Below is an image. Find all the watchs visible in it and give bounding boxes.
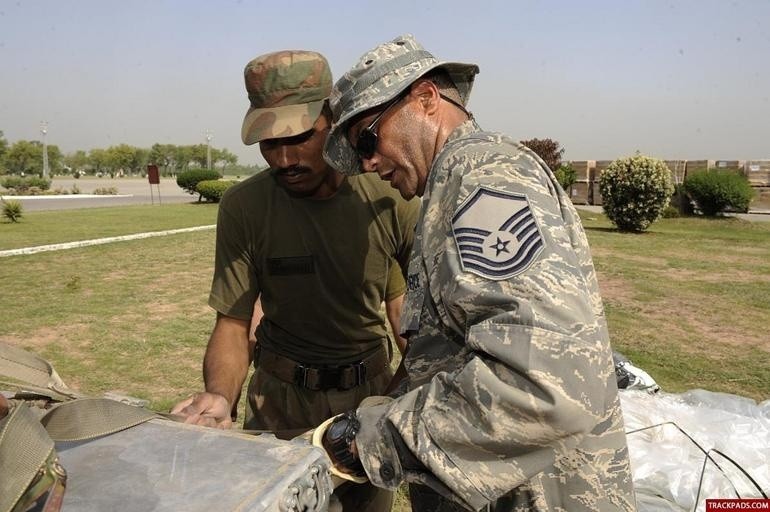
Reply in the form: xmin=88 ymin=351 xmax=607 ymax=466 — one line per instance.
xmin=327 ymin=408 xmax=366 ymax=477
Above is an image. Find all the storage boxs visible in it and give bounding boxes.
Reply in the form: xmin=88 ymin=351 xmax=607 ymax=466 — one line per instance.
xmin=560 ymin=159 xmax=770 ymax=214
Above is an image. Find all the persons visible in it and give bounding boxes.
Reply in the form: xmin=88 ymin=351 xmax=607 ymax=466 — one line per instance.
xmin=167 ymin=51 xmax=423 ymax=512
xmin=290 ymin=34 xmax=634 ymax=512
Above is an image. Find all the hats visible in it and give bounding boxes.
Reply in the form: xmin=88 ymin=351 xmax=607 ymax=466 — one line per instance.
xmin=322 ymin=34 xmax=479 ymax=177
xmin=241 ymin=51 xmax=332 ymax=145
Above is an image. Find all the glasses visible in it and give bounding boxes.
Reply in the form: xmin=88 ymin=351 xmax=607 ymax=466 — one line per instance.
xmin=356 ymin=88 xmax=411 ymax=160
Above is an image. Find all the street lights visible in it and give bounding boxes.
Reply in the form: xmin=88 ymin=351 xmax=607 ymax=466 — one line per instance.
xmin=206 ymin=128 xmax=212 ymax=171
xmin=40 ymin=120 xmax=50 ymax=181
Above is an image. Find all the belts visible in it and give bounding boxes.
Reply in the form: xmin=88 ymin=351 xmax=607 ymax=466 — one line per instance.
xmin=257 ymin=346 xmax=390 ymax=391
